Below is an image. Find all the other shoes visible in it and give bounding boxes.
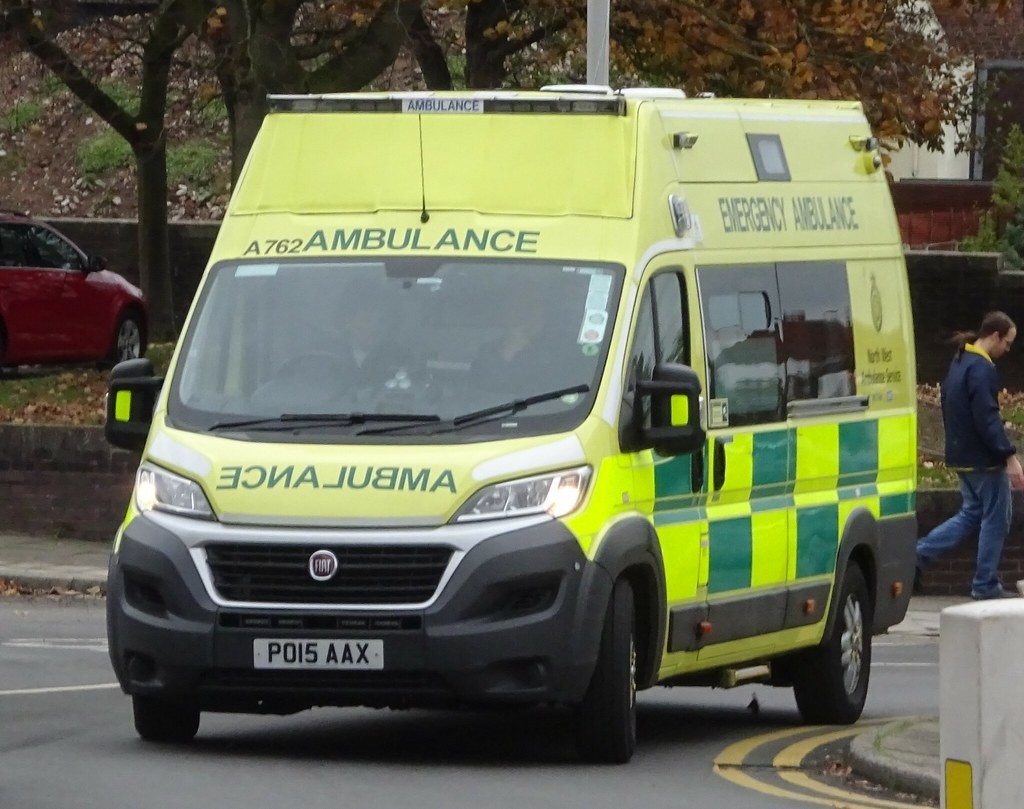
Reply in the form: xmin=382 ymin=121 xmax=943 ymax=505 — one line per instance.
xmin=913 ymin=566 xmax=926 ymax=593
xmin=971 ymin=589 xmax=1019 ymax=600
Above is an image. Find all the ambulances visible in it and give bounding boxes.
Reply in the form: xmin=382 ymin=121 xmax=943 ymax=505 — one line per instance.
xmin=104 ymin=80 xmax=922 ymax=764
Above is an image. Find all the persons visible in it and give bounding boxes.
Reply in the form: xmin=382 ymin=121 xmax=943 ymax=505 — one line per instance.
xmin=333 ymin=304 xmax=408 ymax=395
xmin=916 ymin=312 xmax=1024 ymax=600
xmin=470 ymin=281 xmax=558 ymax=403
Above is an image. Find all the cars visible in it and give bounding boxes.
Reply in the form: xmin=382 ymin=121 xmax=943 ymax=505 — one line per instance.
xmin=0 ymin=206 xmax=146 ymax=374
